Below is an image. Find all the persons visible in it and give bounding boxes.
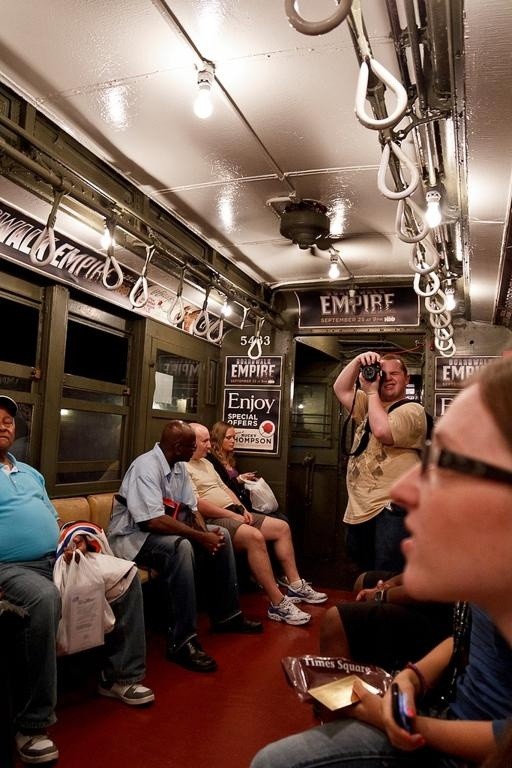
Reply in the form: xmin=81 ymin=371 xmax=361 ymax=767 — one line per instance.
xmin=184 ymin=421 xmax=330 ymax=628
xmin=105 ymin=418 xmax=265 ymax=674
xmin=321 ymin=568 xmax=471 ymax=666
xmin=203 ymin=420 xmax=292 ymax=592
xmin=332 ymin=349 xmax=430 ymax=577
xmin=248 ymin=597 xmax=511 ymax=767
xmin=2 ymin=394 xmax=159 ymax=767
xmin=383 ymin=356 xmax=512 ymax=644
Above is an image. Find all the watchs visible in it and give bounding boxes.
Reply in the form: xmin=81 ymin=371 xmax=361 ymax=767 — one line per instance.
xmin=373 ymin=587 xmax=387 ymax=602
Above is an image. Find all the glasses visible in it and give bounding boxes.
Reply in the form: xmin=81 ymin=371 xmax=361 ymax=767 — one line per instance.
xmin=182 ymin=444 xmax=197 ymax=452
xmin=419 ymin=438 xmax=512 ymax=490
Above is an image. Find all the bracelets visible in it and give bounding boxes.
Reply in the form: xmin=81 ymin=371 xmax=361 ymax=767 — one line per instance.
xmin=405 ymin=660 xmax=428 ymax=697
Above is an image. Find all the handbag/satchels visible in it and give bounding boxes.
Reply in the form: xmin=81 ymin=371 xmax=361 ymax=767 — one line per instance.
xmin=233 ymin=476 xmax=253 ymax=512
xmin=176 ymin=502 xmax=202 ymax=531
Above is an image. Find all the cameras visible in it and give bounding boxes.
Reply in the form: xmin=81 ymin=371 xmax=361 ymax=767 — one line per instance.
xmin=358 ymin=363 xmax=381 ymax=386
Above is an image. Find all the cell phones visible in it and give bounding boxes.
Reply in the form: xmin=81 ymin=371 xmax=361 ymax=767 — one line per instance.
xmin=390 ymin=681 xmax=414 ymax=735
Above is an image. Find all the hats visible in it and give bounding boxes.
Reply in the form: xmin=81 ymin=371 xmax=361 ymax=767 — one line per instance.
xmin=0 ymin=393 xmax=19 ymax=418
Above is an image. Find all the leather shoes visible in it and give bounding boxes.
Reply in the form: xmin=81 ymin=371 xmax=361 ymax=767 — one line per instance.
xmin=209 ymin=618 xmax=264 ymax=634
xmin=167 ymin=637 xmax=218 ymax=673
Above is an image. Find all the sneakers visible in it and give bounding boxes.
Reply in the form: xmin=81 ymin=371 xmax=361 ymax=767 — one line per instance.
xmin=97 ymin=670 xmax=155 ymax=705
xmin=15 ymin=731 xmax=59 ymax=764
xmin=285 ymin=578 xmax=328 ymax=604
xmin=267 ymin=594 xmax=312 ymax=625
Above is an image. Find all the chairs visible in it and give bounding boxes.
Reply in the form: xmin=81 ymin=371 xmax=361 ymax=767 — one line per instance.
xmin=47 ymin=491 xmax=161 ymax=611
